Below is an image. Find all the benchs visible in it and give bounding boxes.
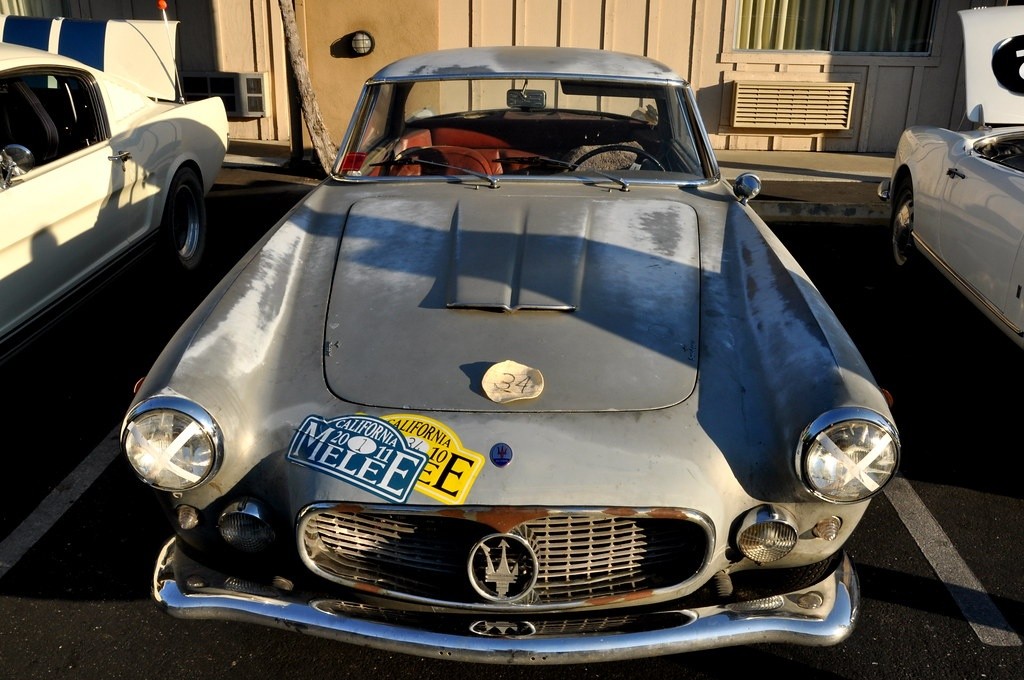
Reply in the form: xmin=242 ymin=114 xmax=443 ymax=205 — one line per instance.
xmin=393 ymin=118 xmax=660 ymax=174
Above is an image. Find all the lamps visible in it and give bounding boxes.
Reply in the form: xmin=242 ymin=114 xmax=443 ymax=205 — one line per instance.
xmin=352 ymin=30 xmax=375 ymax=55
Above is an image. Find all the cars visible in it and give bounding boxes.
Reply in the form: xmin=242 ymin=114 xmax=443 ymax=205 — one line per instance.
xmin=875 ymin=4 xmax=1024 ymax=357
xmin=0 ymin=41 xmax=232 ymax=350
xmin=118 ymin=38 xmax=903 ymax=670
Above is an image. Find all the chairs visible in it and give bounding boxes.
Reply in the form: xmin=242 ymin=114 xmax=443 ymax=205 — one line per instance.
xmin=565 ymin=141 xmax=645 ymax=172
xmin=390 ymin=146 xmax=492 ymax=176
